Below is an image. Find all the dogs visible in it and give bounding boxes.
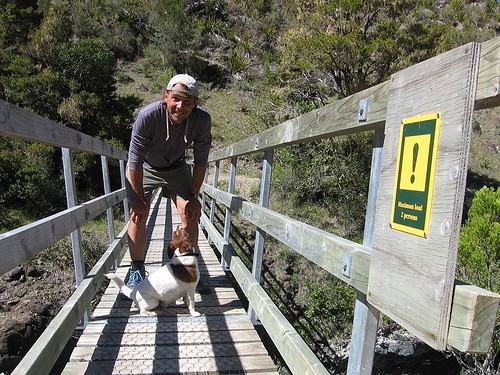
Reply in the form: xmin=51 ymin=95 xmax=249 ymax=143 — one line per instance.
xmin=103 ymin=223 xmax=201 ymax=317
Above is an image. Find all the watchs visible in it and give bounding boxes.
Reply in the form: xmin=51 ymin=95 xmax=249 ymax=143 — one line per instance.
xmin=190 ymin=192 xmax=198 ymax=198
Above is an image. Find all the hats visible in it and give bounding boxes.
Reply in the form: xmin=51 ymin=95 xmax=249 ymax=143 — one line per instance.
xmin=166 ymin=74 xmax=200 ymax=99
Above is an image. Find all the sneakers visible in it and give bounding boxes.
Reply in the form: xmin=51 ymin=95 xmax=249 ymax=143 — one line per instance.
xmin=198 ymin=277 xmax=212 ymax=295
xmin=121 ymin=267 xmax=145 ymax=302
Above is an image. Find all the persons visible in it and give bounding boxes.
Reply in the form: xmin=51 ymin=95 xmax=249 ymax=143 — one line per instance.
xmin=123 ymin=75 xmax=212 ymax=293
